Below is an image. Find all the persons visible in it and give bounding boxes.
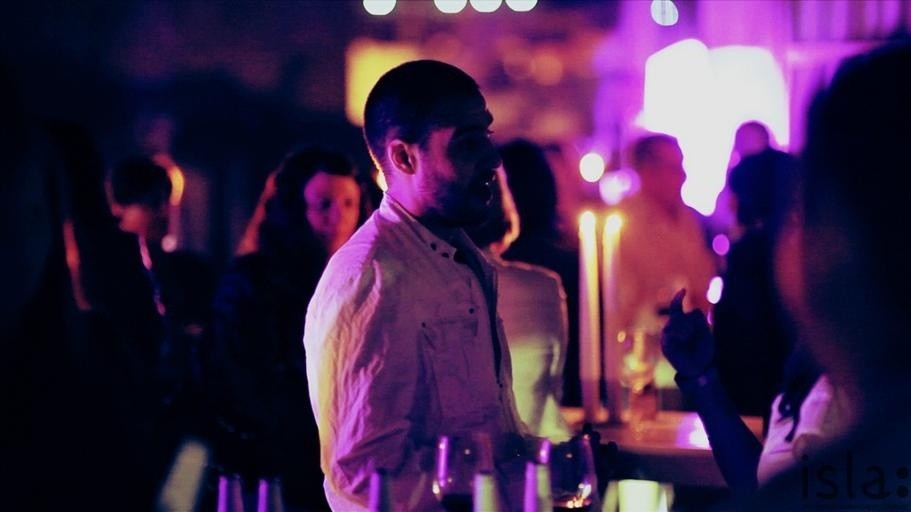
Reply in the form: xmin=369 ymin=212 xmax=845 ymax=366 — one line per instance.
xmin=2 ymin=21 xmax=908 ymax=510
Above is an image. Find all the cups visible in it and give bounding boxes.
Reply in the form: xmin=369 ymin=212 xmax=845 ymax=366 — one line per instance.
xmin=433 ymin=431 xmax=493 ymax=511
xmin=213 ymin=474 xmax=285 ymax=512
xmin=523 ymin=432 xmax=600 ymax=512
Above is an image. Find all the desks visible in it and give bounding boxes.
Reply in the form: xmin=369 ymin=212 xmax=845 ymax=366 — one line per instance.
xmin=555 ymin=404 xmax=766 ymax=512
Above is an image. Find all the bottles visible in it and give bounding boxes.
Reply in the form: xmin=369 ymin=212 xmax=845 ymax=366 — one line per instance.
xmin=618 ymin=325 xmax=657 ymax=423
